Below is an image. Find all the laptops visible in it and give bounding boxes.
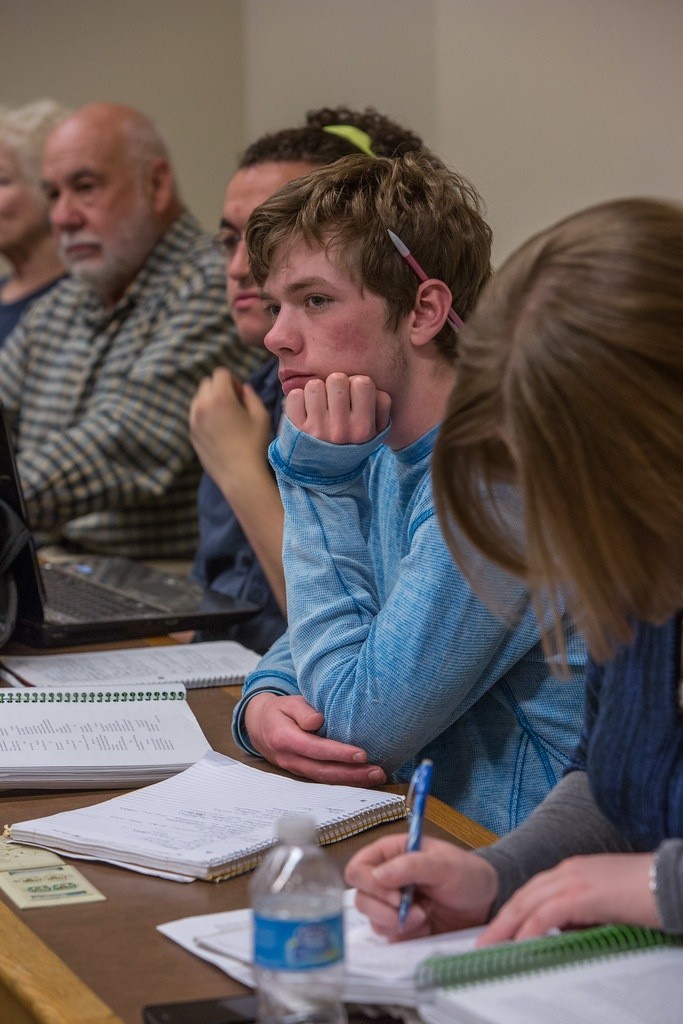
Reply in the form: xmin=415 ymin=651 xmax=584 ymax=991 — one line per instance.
xmin=0 ymin=408 xmax=262 ymax=650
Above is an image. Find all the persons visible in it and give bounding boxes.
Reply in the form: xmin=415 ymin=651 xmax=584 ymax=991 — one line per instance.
xmin=344 ymin=198 xmax=683 ymax=944
xmin=0 ymin=100 xmax=273 ymax=560
xmin=233 ymin=157 xmax=587 ymax=841
xmin=186 ymin=96 xmax=432 ymax=657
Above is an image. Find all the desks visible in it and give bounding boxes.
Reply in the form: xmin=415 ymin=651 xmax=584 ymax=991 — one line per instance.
xmin=2 ymin=631 xmax=503 ymax=1023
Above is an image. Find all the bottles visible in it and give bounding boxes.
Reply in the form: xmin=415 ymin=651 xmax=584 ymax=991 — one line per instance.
xmin=248 ymin=817 xmax=348 ymax=1024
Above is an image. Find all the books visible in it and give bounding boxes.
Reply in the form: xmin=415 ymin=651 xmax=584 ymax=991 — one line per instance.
xmin=1 ymin=640 xmax=683 ymax=1024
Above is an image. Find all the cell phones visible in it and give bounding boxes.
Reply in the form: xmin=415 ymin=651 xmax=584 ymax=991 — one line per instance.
xmin=142 ymin=992 xmax=318 ymax=1024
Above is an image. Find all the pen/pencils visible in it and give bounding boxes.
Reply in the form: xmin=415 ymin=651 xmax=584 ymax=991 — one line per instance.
xmin=398 ymin=758 xmax=434 ymax=926
xmin=388 ymin=228 xmax=465 ymax=334
